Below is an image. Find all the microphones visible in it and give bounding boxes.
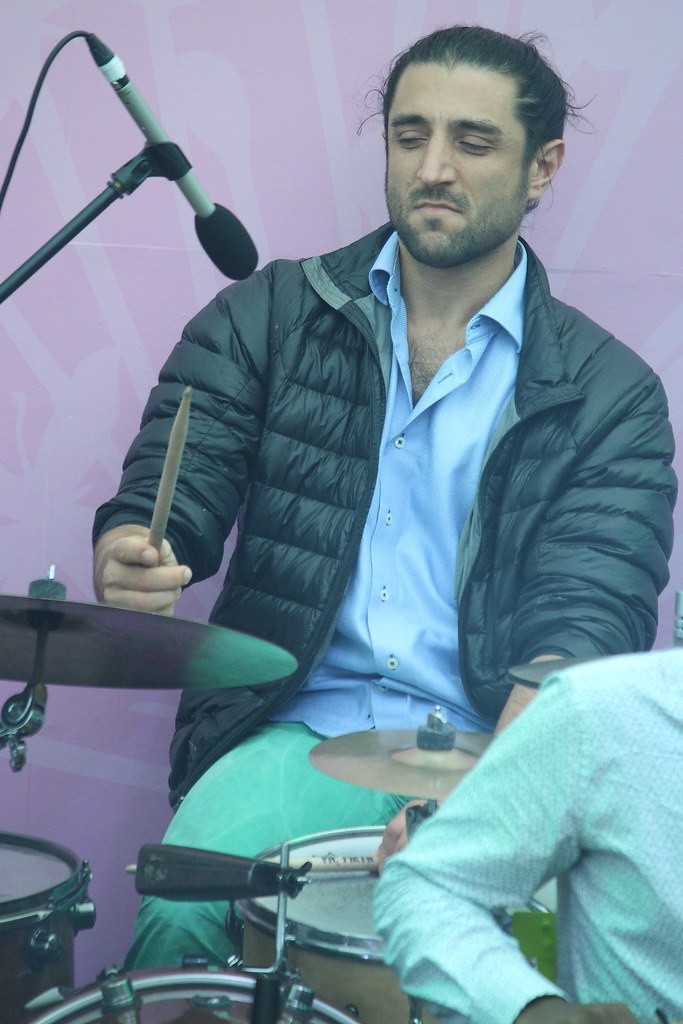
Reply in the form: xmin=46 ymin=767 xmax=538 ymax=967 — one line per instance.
xmin=85 ymin=33 xmax=259 ymax=281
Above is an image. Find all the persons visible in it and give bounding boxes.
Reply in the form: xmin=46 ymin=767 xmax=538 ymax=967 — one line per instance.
xmin=372 ymin=647 xmax=683 ymax=1024
xmin=90 ymin=25 xmax=676 ymax=971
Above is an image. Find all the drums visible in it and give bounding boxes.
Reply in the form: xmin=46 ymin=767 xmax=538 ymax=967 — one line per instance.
xmin=19 ymin=965 xmax=362 ymax=1024
xmin=0 ymin=829 xmax=96 ymax=1024
xmin=237 ymin=825 xmax=553 ymax=1024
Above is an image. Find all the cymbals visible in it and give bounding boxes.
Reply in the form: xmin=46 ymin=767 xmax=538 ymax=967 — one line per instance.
xmin=0 ymin=593 xmax=300 ymax=691
xmin=307 ymin=726 xmax=498 ymax=802
xmin=507 ymin=653 xmax=608 ymax=690
